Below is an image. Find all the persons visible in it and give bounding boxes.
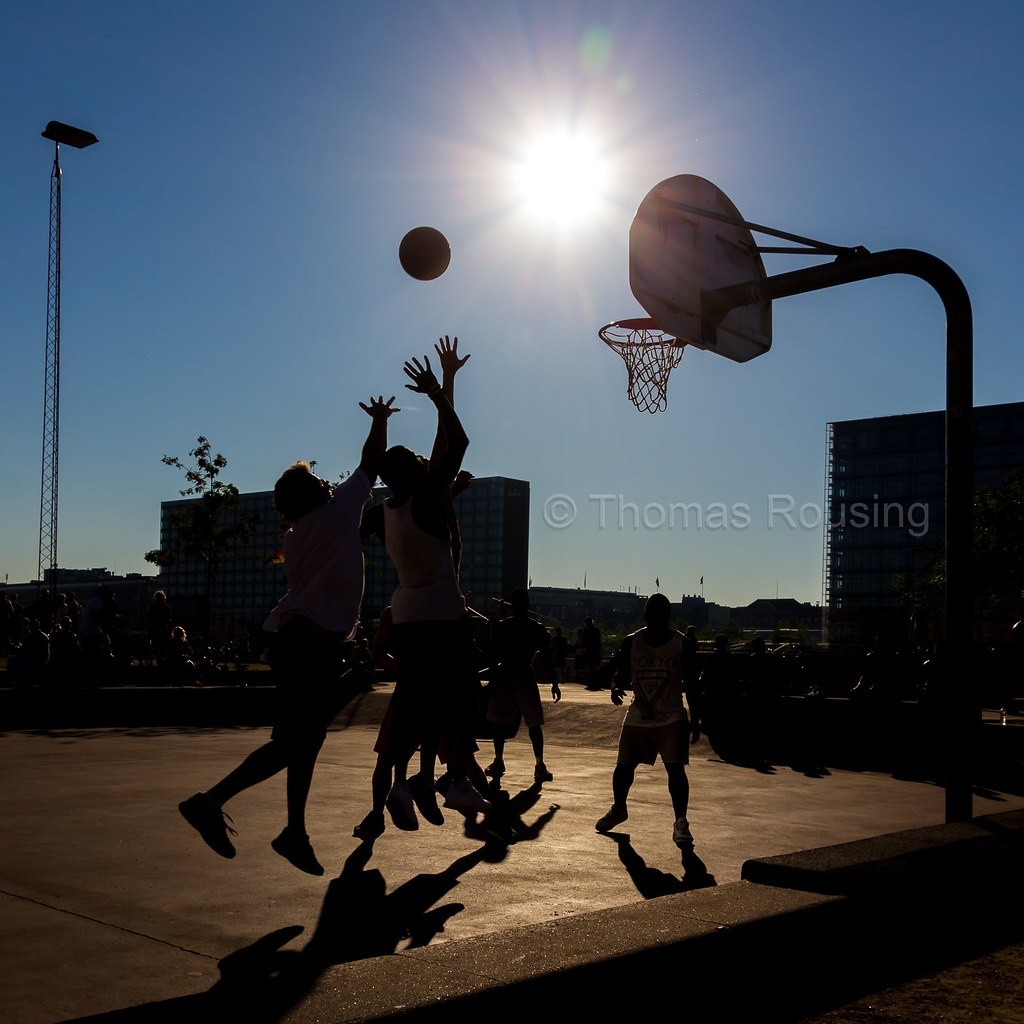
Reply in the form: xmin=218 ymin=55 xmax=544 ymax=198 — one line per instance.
xmin=178 ymin=333 xmax=561 ymax=874
xmin=0 ymin=585 xmax=206 ymax=687
xmin=681 ymin=622 xmax=907 ymax=746
xmin=546 ymin=613 xmax=607 ymax=692
xmin=597 ymin=594 xmax=701 ymax=842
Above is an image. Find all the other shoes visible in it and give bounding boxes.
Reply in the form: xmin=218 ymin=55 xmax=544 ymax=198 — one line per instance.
xmin=353 ymin=812 xmax=385 ymax=836
xmin=675 ymin=817 xmax=693 ymax=846
xmin=596 ymin=804 xmax=628 ymax=832
xmin=485 ymin=759 xmax=505 ymax=776
xmin=271 ymin=828 xmax=324 ymax=875
xmin=535 ymin=767 xmax=552 ymax=781
xmin=406 ymin=772 xmax=444 ymax=825
xmin=435 ymin=774 xmax=475 ymax=817
xmin=179 ymin=793 xmax=238 ymax=859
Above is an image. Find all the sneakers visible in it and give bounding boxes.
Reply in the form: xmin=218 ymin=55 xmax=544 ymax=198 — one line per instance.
xmin=443 ymin=781 xmax=492 ymax=812
xmin=386 ymin=783 xmax=418 ymax=831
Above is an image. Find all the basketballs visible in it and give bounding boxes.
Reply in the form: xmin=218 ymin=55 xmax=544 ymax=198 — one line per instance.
xmin=398 ymin=226 xmax=451 ymax=281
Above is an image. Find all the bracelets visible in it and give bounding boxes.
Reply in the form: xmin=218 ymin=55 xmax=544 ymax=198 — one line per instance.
xmin=431 ymin=387 xmax=445 ymax=399
